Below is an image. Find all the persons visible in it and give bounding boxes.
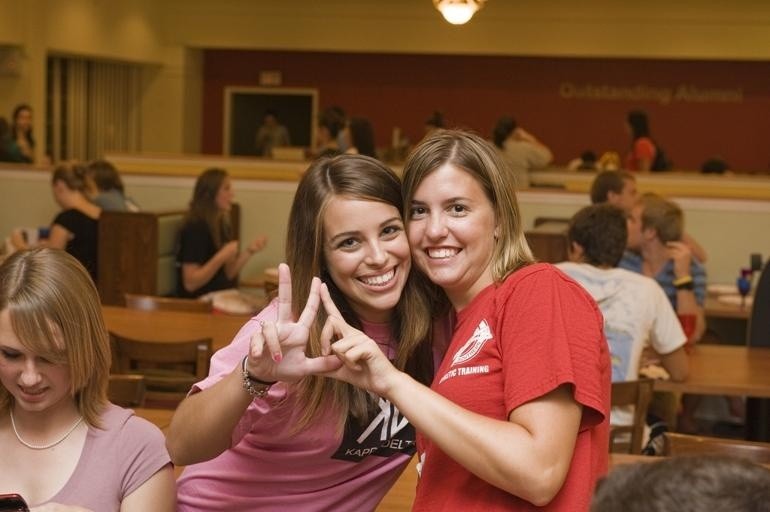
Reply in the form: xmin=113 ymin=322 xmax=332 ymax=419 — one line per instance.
xmin=427 ymin=115 xmax=445 ymax=132
xmin=166 ymin=153 xmax=459 ymax=512
xmin=489 ymin=115 xmax=554 ymax=192
xmin=312 ymin=128 xmax=612 ymax=512
xmin=549 ymin=149 xmax=729 ymax=459
xmin=0 ymin=245 xmax=175 ymax=512
xmin=0 ymin=105 xmax=35 ymax=163
xmin=85 ymin=159 xmax=143 ymax=214
xmin=0 ymin=115 xmax=12 ymax=161
xmin=174 ymin=168 xmax=268 ymax=299
xmin=310 ymin=110 xmax=377 ymax=163
xmin=621 ymin=109 xmax=658 ymax=172
xmin=589 ymin=449 xmax=770 ymax=512
xmin=11 ymin=162 xmax=104 ymax=285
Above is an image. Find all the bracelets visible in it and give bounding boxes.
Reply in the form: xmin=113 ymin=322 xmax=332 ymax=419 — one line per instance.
xmin=242 ymin=357 xmax=275 ymax=402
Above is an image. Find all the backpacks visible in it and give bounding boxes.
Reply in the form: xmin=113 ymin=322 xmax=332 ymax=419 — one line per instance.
xmin=646 ymin=135 xmax=674 ymax=172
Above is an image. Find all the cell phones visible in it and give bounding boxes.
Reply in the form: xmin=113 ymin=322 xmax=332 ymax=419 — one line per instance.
xmin=0 ymin=492 xmax=30 ymax=512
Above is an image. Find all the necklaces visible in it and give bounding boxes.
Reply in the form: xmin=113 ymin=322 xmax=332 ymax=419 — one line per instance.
xmin=7 ymin=407 xmax=85 ymax=449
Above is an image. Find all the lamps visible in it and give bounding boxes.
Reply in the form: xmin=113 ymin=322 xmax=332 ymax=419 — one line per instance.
xmin=431 ymin=0 xmax=484 ymax=27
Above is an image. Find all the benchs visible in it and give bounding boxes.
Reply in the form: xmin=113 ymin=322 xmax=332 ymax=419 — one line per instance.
xmin=523 ymin=213 xmax=571 ymax=262
xmin=93 ymin=201 xmax=242 ymax=308
